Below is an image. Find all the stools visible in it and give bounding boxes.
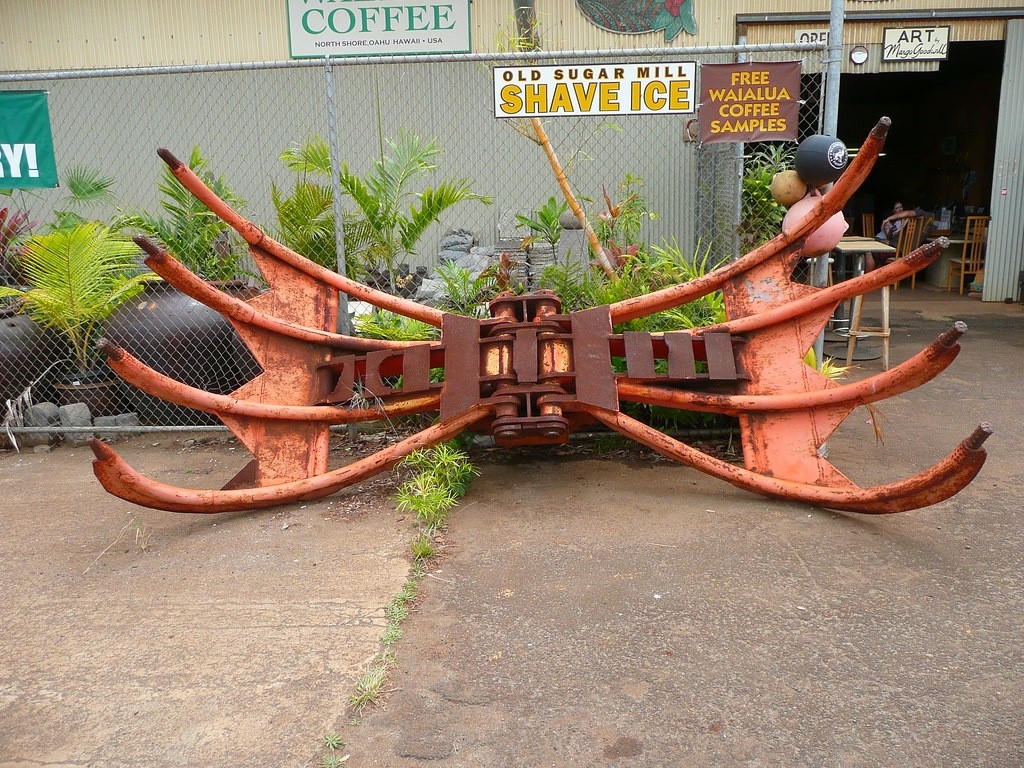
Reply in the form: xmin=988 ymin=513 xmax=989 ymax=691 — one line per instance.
xmin=824 ymin=235 xmax=895 ymax=372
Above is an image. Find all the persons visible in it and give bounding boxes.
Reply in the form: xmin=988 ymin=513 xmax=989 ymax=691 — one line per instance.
xmin=865 ymin=201 xmax=933 ymax=274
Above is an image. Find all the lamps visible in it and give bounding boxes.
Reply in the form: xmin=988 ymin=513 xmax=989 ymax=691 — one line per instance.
xmin=850 ymin=44 xmax=868 ymax=64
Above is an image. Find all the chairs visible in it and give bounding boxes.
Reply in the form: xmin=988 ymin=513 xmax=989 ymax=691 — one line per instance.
xmin=886 ymin=216 xmax=925 ymax=290
xmin=862 ymin=214 xmax=875 ymax=239
xmin=948 ymin=215 xmax=990 ymax=293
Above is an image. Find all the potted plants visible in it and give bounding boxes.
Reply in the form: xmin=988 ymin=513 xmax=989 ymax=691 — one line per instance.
xmin=0 ymin=145 xmax=265 ymax=426
